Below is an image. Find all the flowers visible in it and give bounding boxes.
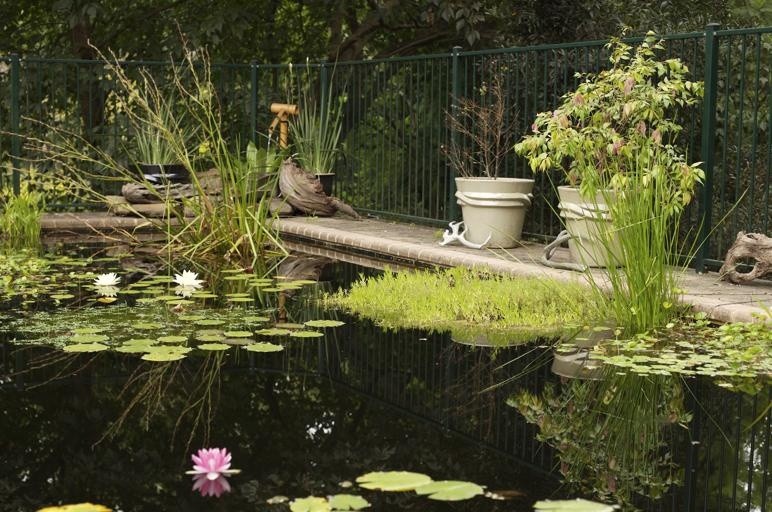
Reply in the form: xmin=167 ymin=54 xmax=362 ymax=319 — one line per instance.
xmin=94 ymin=285 xmax=121 ymax=298
xmin=191 ymin=476 xmax=233 ymax=499
xmin=93 ymin=271 xmax=122 ymax=286
xmin=184 ymin=446 xmax=244 ymax=480
xmin=171 ymin=269 xmax=207 ymax=288
xmin=174 ymin=285 xmax=196 ymax=298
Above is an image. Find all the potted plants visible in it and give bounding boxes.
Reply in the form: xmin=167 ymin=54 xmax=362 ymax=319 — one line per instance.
xmin=123 ymin=71 xmax=220 ymax=185
xmin=514 ymin=28 xmax=707 ymax=271
xmin=435 ymin=330 xmax=536 ymax=441
xmin=506 ymin=377 xmax=685 ymax=503
xmin=256 ymin=47 xmax=349 ymax=197
xmin=282 ymin=264 xmax=346 ymax=393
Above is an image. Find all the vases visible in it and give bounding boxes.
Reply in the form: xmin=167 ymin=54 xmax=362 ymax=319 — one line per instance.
xmin=548 ymin=325 xmax=616 ymax=381
xmin=453 ymin=176 xmax=534 ymax=248
